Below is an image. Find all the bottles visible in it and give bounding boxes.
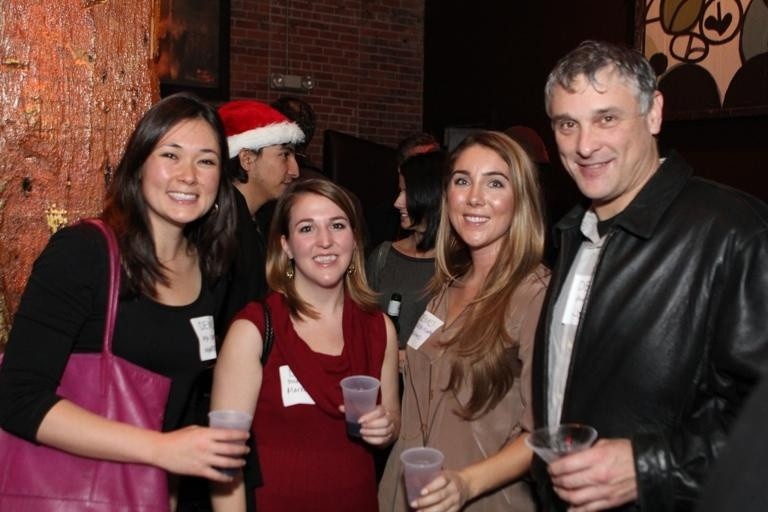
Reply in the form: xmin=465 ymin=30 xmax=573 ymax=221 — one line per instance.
xmin=385 ymin=293 xmax=402 ymax=336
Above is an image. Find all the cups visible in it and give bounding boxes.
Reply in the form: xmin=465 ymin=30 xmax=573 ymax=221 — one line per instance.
xmin=400 ymin=447 xmax=445 ymax=507
xmin=207 ymin=409 xmax=253 ymax=479
xmin=524 ymin=422 xmax=598 ymax=467
xmin=341 ymin=374 xmax=381 ymax=441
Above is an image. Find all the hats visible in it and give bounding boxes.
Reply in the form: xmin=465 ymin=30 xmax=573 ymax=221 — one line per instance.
xmin=216 ymin=99 xmax=307 ymax=160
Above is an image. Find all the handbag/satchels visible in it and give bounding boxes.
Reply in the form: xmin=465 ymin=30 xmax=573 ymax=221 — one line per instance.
xmin=186 ymin=365 xmax=263 ymax=487
xmin=2 ymin=350 xmax=173 ymax=512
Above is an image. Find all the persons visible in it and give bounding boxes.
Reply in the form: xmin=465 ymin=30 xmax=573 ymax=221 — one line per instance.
xmin=530 ymin=37 xmax=768 ymax=512
xmin=0 ymin=92 xmax=250 ymax=512
xmin=176 ymin=99 xmax=305 ymax=512
xmin=365 ymin=152 xmax=468 ymax=371
xmin=375 ymin=129 xmax=554 ymax=512
xmin=210 ymin=178 xmax=402 ymax=512
